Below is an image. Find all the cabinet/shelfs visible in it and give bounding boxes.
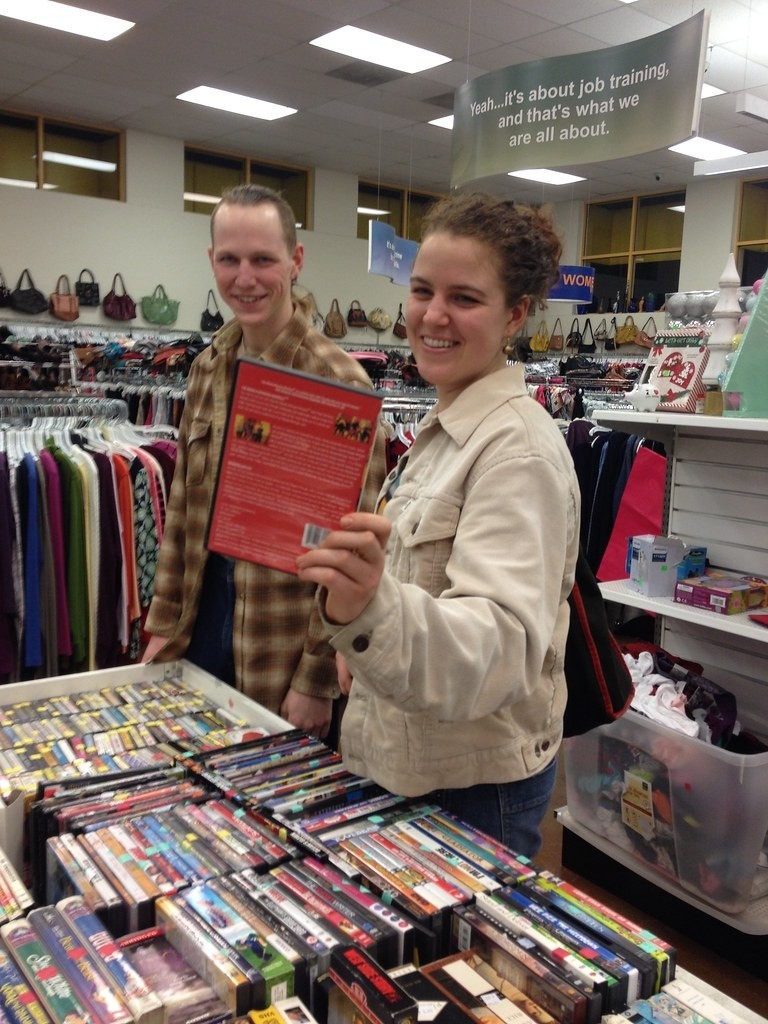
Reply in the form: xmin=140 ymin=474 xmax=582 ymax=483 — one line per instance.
xmin=554 ymin=409 xmax=768 ymax=935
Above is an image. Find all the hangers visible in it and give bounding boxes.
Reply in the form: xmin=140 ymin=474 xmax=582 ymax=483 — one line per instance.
xmin=346 ymin=344 xmax=648 ymax=448
xmin=0 ymin=326 xmax=210 ymax=466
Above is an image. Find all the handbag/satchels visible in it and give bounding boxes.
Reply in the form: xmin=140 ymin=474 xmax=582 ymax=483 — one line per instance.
xmin=199 ymin=289 xmax=225 ymax=331
xmin=529 ymin=320 xmax=550 ymax=353
xmin=565 ymin=318 xmax=582 ymax=347
xmin=634 ymin=317 xmax=658 ymax=349
xmin=593 ymin=319 xmax=607 ymax=341
xmin=578 ymin=318 xmax=597 ymax=353
xmin=548 ymin=317 xmax=564 ymax=351
xmin=10 ymin=269 xmax=50 ymax=315
xmin=604 ymin=316 xmax=620 ymax=351
xmin=516 ymin=320 xmax=532 ymax=353
xmin=557 ymin=354 xmax=591 ymax=375
xmin=311 ymin=313 xmax=325 ymax=334
xmin=141 ymin=284 xmax=181 ymax=326
xmin=324 ymin=299 xmax=348 ymax=338
xmin=103 ymin=272 xmax=137 ymax=320
xmin=614 ymin=315 xmax=640 ymax=345
xmin=348 ymin=299 xmax=368 ymax=328
xmin=562 ymin=540 xmax=636 ymax=740
xmin=74 ymin=269 xmax=100 ymax=307
xmin=393 ymin=303 xmax=407 ymax=339
xmin=367 ymin=307 xmax=393 ymax=332
xmin=0 ymin=273 xmax=11 ymax=308
xmin=50 ymin=275 xmax=80 ymax=322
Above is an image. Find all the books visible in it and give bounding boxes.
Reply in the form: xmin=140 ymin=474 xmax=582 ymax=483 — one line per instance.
xmin=0 ymin=677 xmax=765 ymax=1024
xmin=202 ymin=356 xmax=384 ymax=583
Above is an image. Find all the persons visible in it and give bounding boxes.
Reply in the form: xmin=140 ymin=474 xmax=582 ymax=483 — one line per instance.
xmin=291 ymin=190 xmax=583 ymax=869
xmin=140 ymin=184 xmax=385 ymax=750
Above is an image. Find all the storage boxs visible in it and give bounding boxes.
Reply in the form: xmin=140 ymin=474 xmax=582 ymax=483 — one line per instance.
xmin=625 ymin=536 xmax=707 ymax=581
xmin=673 ymin=574 xmax=768 ymax=615
xmin=563 ymin=659 xmax=768 ymax=915
xmin=629 ymin=534 xmax=684 ymax=596
